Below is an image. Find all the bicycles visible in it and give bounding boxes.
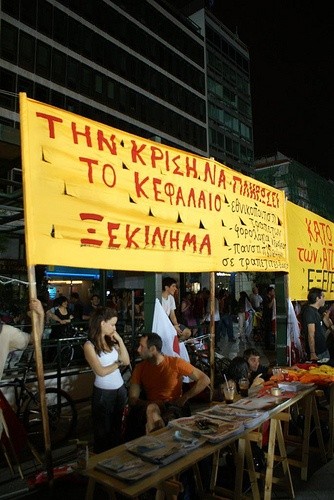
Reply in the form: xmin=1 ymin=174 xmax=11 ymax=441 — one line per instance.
xmin=1 ymin=361 xmax=76 ymax=451
xmin=54 ymin=328 xmax=88 ymax=368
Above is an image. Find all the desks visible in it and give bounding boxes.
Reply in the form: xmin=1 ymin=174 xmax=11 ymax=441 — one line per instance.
xmin=71 ymin=386 xmax=328 ymax=500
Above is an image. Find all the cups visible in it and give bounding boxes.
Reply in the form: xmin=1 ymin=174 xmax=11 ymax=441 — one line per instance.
xmin=223 ymin=387 xmax=234 ymax=404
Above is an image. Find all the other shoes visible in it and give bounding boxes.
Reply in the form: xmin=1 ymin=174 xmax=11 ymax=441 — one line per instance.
xmin=243 ymin=460 xmax=266 ymax=473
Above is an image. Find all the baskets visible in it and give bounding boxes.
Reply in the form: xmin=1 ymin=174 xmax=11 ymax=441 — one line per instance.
xmin=63 ymin=327 xmax=79 ymax=341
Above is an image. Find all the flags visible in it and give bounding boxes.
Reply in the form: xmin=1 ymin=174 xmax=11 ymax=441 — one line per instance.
xmin=150 ymin=297 xmax=196 ymax=384
xmin=287 ymin=299 xmax=307 ymax=366
xmin=0 ymin=389 xmax=42 ymax=479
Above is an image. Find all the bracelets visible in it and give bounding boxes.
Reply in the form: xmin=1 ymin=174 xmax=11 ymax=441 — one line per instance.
xmin=310 ymin=352 xmax=316 ymax=353
xmin=115 ymin=360 xmax=123 ymax=366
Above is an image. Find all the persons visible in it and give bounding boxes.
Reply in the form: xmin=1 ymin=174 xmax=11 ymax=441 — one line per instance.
xmin=0 ymin=277 xmax=277 ymax=363
xmin=301 ymin=288 xmax=334 ymax=411
xmin=0 ymin=298 xmax=44 ymax=387
xmin=130 ymin=333 xmax=212 ymax=434
xmin=222 ymin=357 xmax=270 ymax=446
xmin=243 ymin=348 xmax=299 ymax=387
xmin=84 ymin=307 xmax=131 ymax=453
